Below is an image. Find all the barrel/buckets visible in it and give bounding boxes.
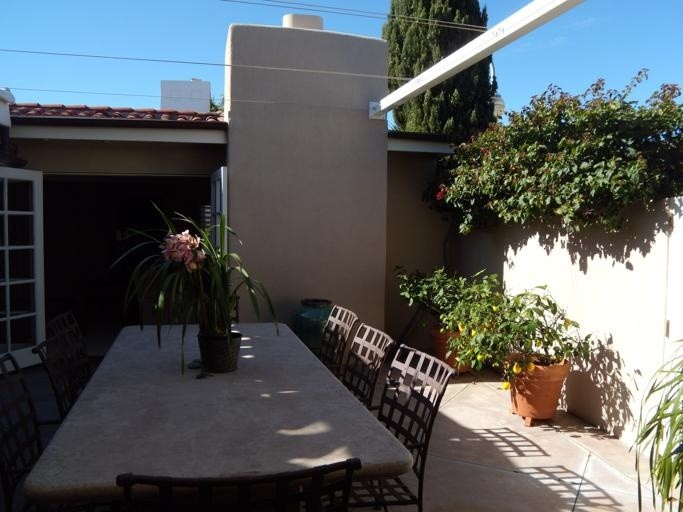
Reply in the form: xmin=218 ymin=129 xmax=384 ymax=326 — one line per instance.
xmin=295 ymin=298 xmax=333 ymax=343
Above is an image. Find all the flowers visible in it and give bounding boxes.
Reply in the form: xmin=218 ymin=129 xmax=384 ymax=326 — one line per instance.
xmin=109 ymin=201 xmax=280 ymax=376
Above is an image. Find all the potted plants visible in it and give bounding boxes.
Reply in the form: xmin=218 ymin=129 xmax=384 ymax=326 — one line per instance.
xmin=393 ymin=263 xmax=592 ymax=428
